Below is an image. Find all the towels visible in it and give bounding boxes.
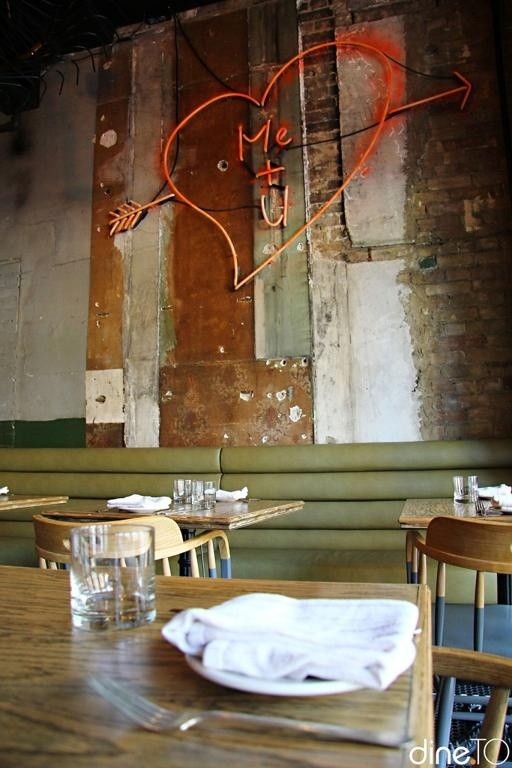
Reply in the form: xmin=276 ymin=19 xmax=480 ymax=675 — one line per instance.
xmin=493 ymin=483 xmax=511 ymax=501
xmin=0 ymin=486 xmax=10 ymax=495
xmin=205 ymin=486 xmax=248 ymax=503
xmin=161 ymin=591 xmax=418 ymax=693
xmin=500 ymin=501 xmax=512 ymax=512
xmin=476 ymin=483 xmax=499 ymax=499
xmin=106 ymin=493 xmax=172 ymax=513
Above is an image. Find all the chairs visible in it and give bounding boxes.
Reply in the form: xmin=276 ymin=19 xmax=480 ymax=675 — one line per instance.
xmin=31 ymin=514 xmax=231 ymax=578
xmin=432 ymin=646 xmax=512 ymax=768
xmin=405 ymin=516 xmax=512 ymax=690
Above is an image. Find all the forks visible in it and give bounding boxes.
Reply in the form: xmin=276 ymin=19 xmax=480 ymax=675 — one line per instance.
xmin=475 ymin=500 xmax=485 ymax=516
xmin=85 ymin=670 xmax=413 ymax=749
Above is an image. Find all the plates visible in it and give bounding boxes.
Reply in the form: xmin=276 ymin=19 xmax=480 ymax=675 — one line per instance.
xmin=119 ymin=508 xmax=169 ymax=514
xmin=479 ymin=496 xmax=492 ymax=499
xmin=183 ymin=653 xmax=364 ymax=697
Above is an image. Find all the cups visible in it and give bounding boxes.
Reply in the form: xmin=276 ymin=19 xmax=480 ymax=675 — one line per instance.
xmin=184 ymin=480 xmax=191 ymax=511
xmin=453 ymin=475 xmax=479 ymax=505
xmin=203 ymin=481 xmax=216 ymax=510
xmin=172 ymin=479 xmax=185 ymax=511
xmin=69 ymin=522 xmax=157 ymax=634
xmin=191 ymin=480 xmax=203 ymax=511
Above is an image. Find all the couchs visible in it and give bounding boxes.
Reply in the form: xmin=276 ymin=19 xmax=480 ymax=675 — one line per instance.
xmin=0 ymin=440 xmax=512 ymax=605
